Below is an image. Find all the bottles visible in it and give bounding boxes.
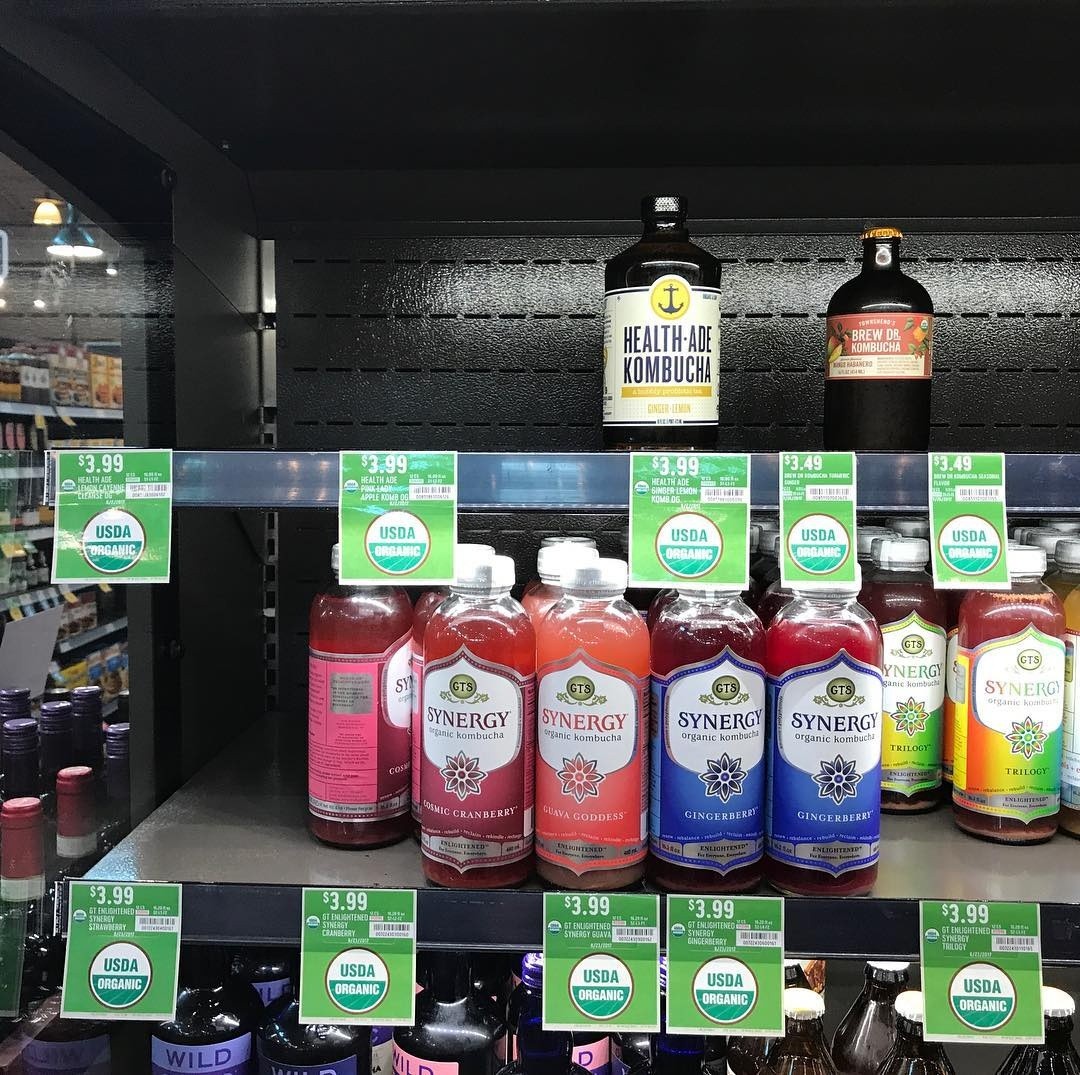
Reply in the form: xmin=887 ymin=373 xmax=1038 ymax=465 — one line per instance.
xmin=822 ymin=225 xmax=936 ymax=453
xmin=600 ymin=187 xmax=727 ymax=451
xmin=0 ymin=512 xmax=1080 ymax=1075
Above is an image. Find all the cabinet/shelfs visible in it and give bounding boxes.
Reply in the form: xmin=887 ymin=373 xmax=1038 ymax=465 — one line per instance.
xmin=0 ymin=400 xmax=1079 ymax=1075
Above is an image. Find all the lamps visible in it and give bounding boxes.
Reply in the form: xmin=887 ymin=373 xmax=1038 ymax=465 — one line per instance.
xmin=46 ymin=204 xmax=104 ymax=258
xmin=33 ymin=192 xmax=65 ymax=226
